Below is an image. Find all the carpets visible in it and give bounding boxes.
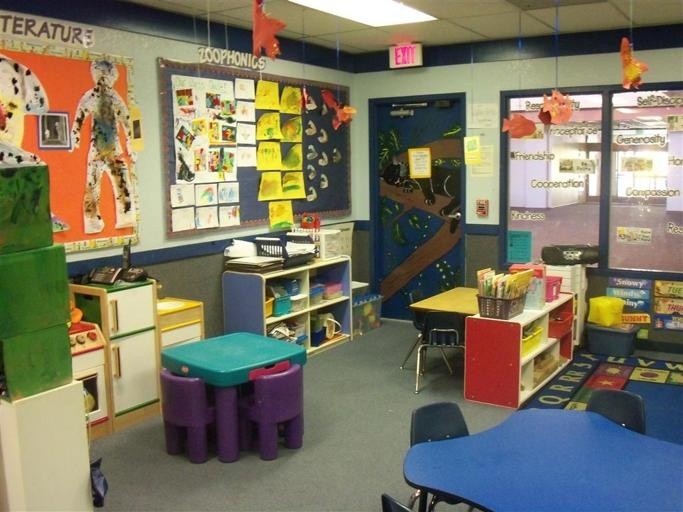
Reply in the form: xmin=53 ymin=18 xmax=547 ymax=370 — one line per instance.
xmin=517 ymin=353 xmax=683 ymax=444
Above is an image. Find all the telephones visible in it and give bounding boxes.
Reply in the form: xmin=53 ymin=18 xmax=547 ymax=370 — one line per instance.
xmin=123 ymin=238 xmax=145 ymax=282
xmin=88 ymin=266 xmax=122 ymax=285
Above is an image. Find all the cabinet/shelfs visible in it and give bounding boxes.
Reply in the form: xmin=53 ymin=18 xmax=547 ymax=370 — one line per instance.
xmin=465 ymin=290 xmax=572 ymax=407
xmin=222 ymin=255 xmax=353 ymax=356
xmin=156 ymin=296 xmax=205 ymax=344
xmin=82 ymin=274 xmax=163 ymax=428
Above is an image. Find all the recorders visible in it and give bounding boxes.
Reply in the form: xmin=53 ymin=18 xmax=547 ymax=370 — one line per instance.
xmin=542 ymin=243 xmax=603 ymax=266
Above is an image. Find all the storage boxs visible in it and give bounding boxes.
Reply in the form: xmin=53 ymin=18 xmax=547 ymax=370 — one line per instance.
xmin=587 ymin=323 xmax=641 ymax=357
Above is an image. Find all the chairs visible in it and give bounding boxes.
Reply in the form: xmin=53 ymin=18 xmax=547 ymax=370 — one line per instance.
xmin=380 ymin=492 xmax=414 ymax=510
xmin=159 ymin=368 xmax=217 ymax=464
xmin=401 ymin=287 xmax=480 ymax=392
xmin=586 ymin=389 xmax=647 ymax=435
xmin=238 ymin=363 xmax=305 ymax=460
xmin=411 ymin=401 xmax=470 ymax=511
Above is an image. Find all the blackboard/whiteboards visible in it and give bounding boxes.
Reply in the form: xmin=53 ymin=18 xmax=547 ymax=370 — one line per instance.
xmin=155 ymin=61 xmax=351 ymax=238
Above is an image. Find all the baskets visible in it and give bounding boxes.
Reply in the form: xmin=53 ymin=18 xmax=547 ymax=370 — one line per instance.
xmin=545 ymin=275 xmax=562 ymax=302
xmin=521 ymin=327 xmax=543 ymax=357
xmin=475 ymin=291 xmax=527 ymax=320
xmin=548 ymin=311 xmax=572 ymax=340
xmin=310 ymin=312 xmax=342 ymax=347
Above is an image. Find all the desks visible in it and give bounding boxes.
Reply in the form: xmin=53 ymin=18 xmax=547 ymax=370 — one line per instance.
xmin=161 ymin=332 xmax=307 ymax=463
xmin=402 ymin=409 xmax=683 ymax=512
